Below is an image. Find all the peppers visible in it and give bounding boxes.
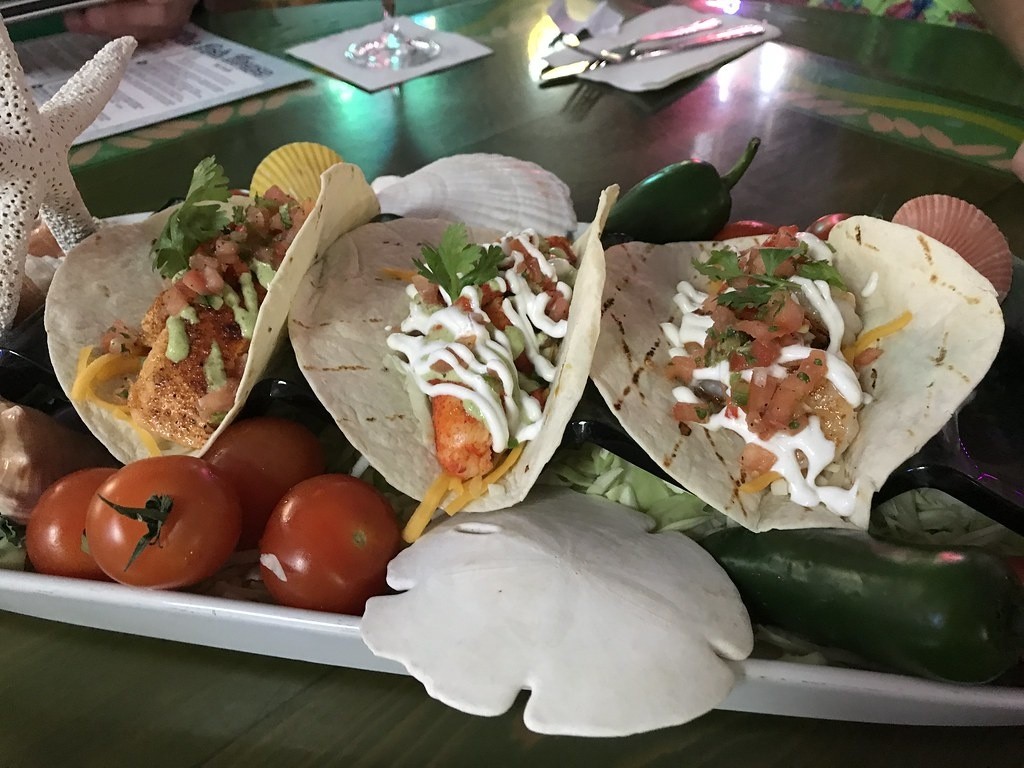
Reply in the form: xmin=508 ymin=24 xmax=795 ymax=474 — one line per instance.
xmin=686 ymin=530 xmax=1024 ymax=684
xmin=598 ymin=136 xmax=761 ymax=248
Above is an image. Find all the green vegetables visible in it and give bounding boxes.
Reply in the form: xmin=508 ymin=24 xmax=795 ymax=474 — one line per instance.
xmin=688 ymin=243 xmax=847 ymax=432
xmin=408 ymin=221 xmax=504 ymax=303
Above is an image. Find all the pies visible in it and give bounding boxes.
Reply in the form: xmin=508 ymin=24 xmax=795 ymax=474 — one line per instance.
xmin=583 ymin=215 xmax=1006 ymax=533
xmin=44 ymin=162 xmax=382 ymax=468
xmin=287 ymin=181 xmax=621 ymax=515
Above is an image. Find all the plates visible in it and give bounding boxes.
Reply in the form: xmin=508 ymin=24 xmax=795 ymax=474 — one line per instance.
xmin=0 ymin=211 xmax=1024 ymax=725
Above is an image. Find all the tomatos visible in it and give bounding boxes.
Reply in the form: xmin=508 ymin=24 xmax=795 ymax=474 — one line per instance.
xmin=85 ymin=455 xmax=242 ymax=591
xmin=261 ymin=473 xmax=401 ymax=615
xmin=24 ymin=468 xmax=123 ymax=582
xmin=197 ymin=418 xmax=323 ymax=543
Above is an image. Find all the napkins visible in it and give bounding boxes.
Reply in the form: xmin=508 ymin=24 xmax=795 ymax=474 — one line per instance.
xmin=542 ymin=5 xmax=781 ymax=93
xmin=286 ymin=14 xmax=493 ymax=92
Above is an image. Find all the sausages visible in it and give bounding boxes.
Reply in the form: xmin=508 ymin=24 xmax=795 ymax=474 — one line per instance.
xmin=427 ymin=372 xmax=493 ymax=479
